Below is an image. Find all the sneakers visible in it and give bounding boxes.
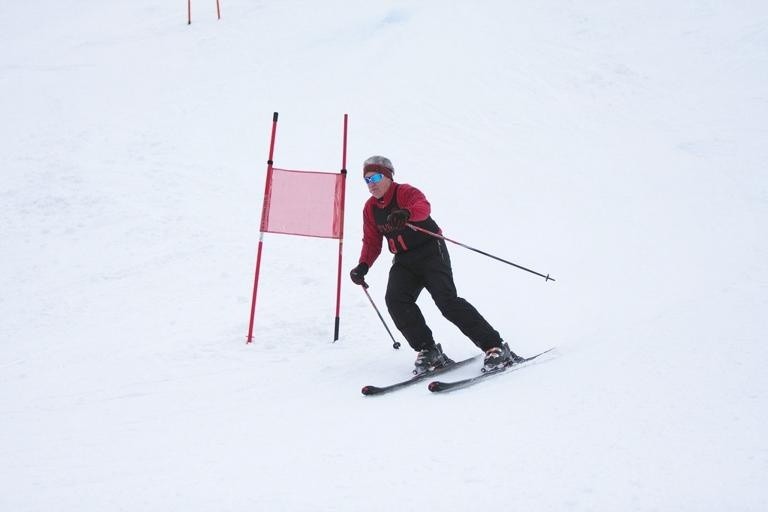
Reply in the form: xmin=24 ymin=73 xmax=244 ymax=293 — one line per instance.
xmin=415 ymin=345 xmax=439 ymax=367
xmin=482 ymin=342 xmax=511 ymax=366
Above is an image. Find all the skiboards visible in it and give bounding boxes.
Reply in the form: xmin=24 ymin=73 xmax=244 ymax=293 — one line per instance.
xmin=361 ymin=347 xmax=556 ymax=396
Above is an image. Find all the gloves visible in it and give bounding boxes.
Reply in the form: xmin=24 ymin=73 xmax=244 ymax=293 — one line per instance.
xmin=349 ymin=262 xmax=369 ymax=288
xmin=387 ymin=209 xmax=410 ymax=227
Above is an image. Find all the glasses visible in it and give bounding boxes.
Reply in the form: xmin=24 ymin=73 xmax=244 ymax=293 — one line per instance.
xmin=364 ymin=174 xmax=384 ymax=184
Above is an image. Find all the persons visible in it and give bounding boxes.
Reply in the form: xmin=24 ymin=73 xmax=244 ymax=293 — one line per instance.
xmin=349 ymin=155 xmax=512 ymax=369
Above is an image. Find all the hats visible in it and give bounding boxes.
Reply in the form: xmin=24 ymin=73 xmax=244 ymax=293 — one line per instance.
xmin=363 ymin=155 xmax=394 ymax=180
